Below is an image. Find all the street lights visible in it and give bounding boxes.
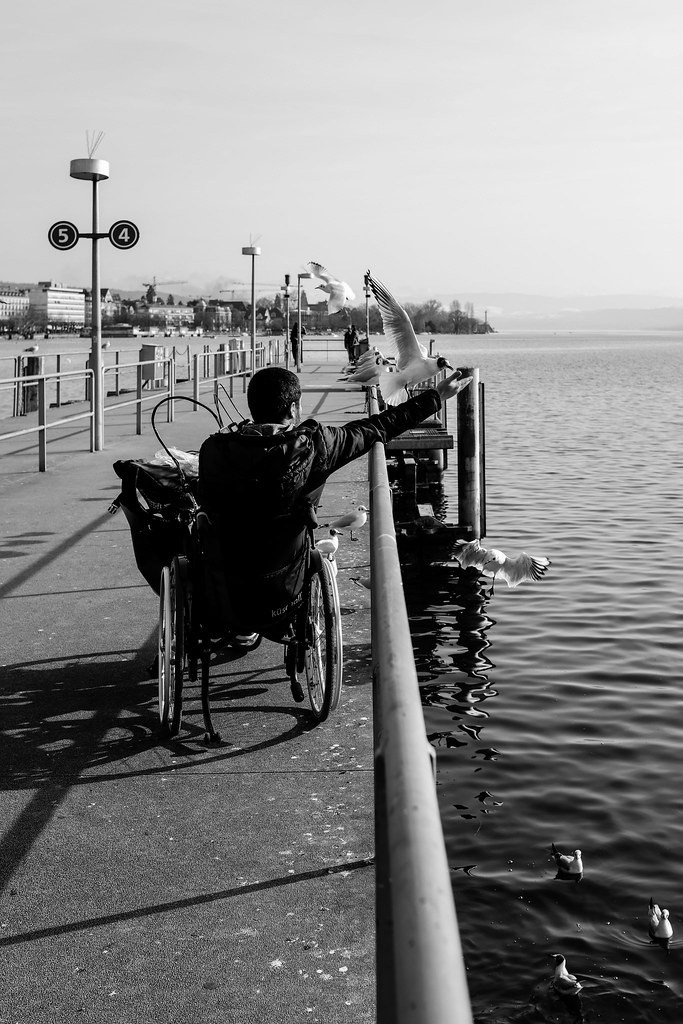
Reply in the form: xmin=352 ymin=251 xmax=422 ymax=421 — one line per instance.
xmin=297 ymin=272 xmax=314 ymax=373
xmin=240 ymin=239 xmax=262 ymax=377
xmin=48 ymin=130 xmax=141 ymax=448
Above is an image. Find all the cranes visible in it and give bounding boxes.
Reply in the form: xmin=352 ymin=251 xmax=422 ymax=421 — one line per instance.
xmin=143 ymin=271 xmax=188 ymax=294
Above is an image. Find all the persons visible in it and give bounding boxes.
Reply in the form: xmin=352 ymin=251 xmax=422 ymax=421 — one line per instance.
xmin=348 ymin=325 xmax=359 ymax=362
xmin=290 ymin=321 xmax=306 ymax=368
xmin=181 ymin=367 xmax=474 ymax=652
xmin=343 ymin=325 xmax=354 ymax=362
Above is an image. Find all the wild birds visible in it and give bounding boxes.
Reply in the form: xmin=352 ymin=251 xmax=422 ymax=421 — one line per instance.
xmin=335 ymin=269 xmax=454 ymax=406
xmin=552 ymin=849 xmax=583 ymax=874
xmin=102 ymin=340 xmax=110 ymax=349
xmin=23 ymin=343 xmax=39 ymax=354
xmin=349 ymin=574 xmax=370 ymax=589
xmin=306 ymin=261 xmax=355 ymax=316
xmin=648 ymin=896 xmax=674 ymax=938
xmin=450 ymin=538 xmax=552 ymax=597
xmin=314 ymin=505 xmax=370 ymax=560
xmin=547 ymin=953 xmax=583 ymax=996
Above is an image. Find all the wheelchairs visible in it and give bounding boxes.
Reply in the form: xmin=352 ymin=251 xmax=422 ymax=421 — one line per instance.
xmin=152 ymin=546 xmax=344 ymax=737
